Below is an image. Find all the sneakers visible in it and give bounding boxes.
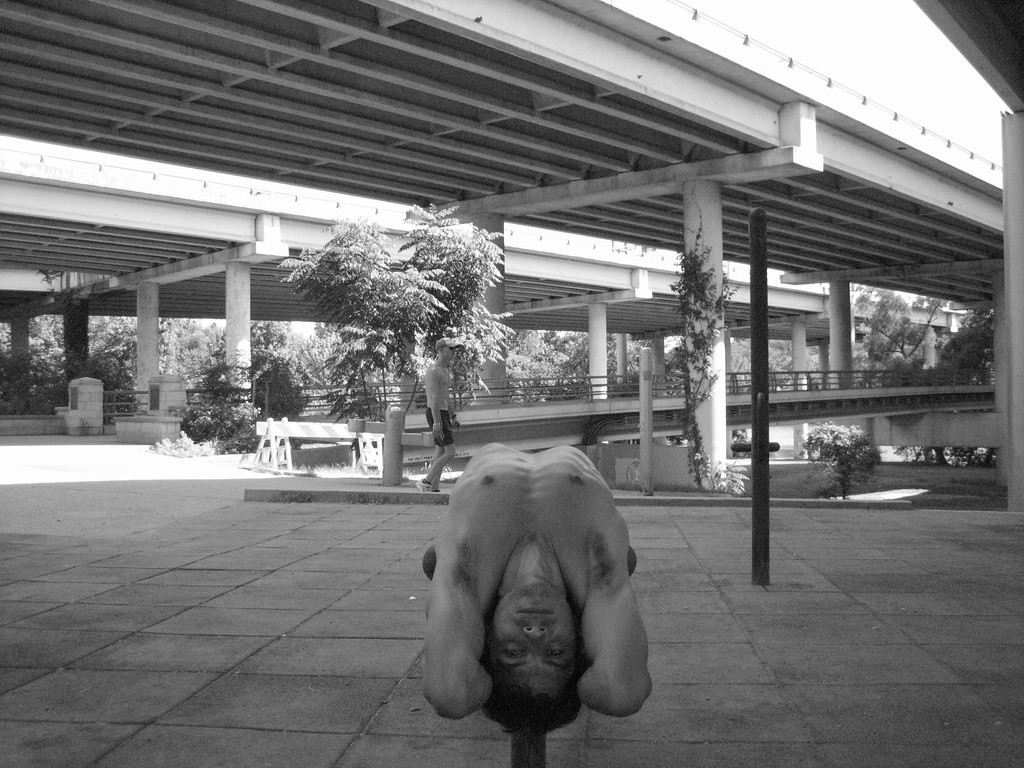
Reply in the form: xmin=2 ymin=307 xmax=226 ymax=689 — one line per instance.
xmin=416 ymin=479 xmax=432 ymax=493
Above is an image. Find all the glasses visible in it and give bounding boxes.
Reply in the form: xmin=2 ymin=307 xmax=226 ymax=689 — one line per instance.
xmin=449 ymin=347 xmax=453 ymax=350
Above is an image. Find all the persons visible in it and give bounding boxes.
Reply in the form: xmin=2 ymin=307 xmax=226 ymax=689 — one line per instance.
xmin=421 ymin=442 xmax=654 ymax=732
xmin=419 ymin=338 xmax=462 ymax=493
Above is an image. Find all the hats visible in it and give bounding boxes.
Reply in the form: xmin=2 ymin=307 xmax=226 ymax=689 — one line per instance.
xmin=436 ymin=338 xmax=457 ymax=350
